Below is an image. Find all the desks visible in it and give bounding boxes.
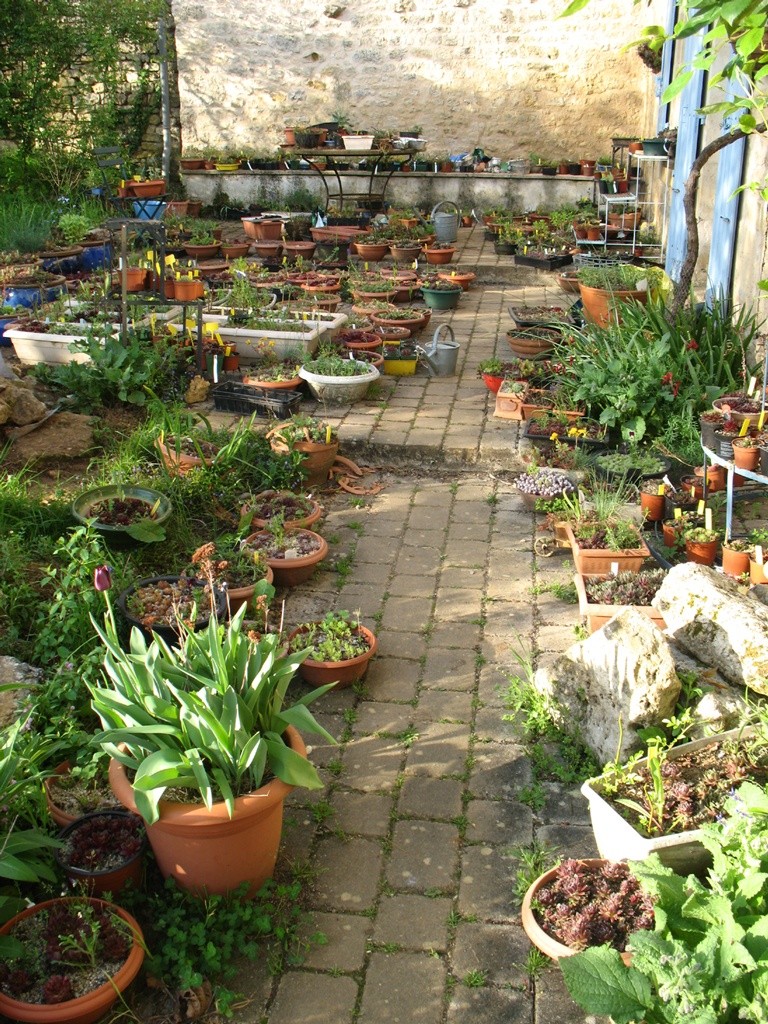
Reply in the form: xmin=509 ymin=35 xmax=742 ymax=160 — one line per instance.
xmin=296 ymin=149 xmax=417 ymax=221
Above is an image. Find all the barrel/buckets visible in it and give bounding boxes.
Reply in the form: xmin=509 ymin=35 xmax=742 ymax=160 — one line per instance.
xmin=132 ymin=201 xmax=167 ymax=220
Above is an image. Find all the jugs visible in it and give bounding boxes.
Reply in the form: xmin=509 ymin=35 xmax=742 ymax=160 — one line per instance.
xmin=412 ymin=200 xmax=461 ymax=244
xmin=420 ymin=324 xmax=460 ymax=378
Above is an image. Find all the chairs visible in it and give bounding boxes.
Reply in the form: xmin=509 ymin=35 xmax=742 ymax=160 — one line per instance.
xmin=93 ymin=146 xmax=171 ymax=249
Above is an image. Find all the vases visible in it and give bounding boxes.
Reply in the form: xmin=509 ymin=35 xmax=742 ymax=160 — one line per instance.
xmin=109 ymin=724 xmax=306 ymax=903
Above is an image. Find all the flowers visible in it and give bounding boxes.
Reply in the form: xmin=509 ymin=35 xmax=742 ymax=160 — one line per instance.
xmin=82 ymin=564 xmax=341 ymax=825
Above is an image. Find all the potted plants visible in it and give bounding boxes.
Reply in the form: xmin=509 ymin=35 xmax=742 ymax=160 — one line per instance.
xmin=0 ymin=121 xmax=768 ymax=1023
